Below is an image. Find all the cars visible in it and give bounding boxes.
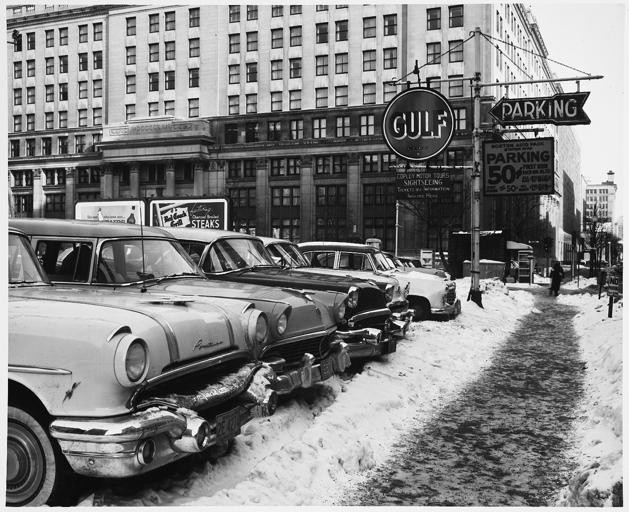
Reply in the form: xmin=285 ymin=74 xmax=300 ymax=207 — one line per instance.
xmin=249 ymin=233 xmax=398 ymax=363
xmin=282 ymin=215 xmax=460 ymax=414
xmin=55 ymin=228 xmax=352 ymax=394
xmin=6 ymin=226 xmax=273 ymax=506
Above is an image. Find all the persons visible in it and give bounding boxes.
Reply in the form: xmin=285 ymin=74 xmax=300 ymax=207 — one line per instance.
xmin=509 ymin=259 xmax=519 ymax=283
xmin=546 ymin=260 xmax=564 ymax=297
